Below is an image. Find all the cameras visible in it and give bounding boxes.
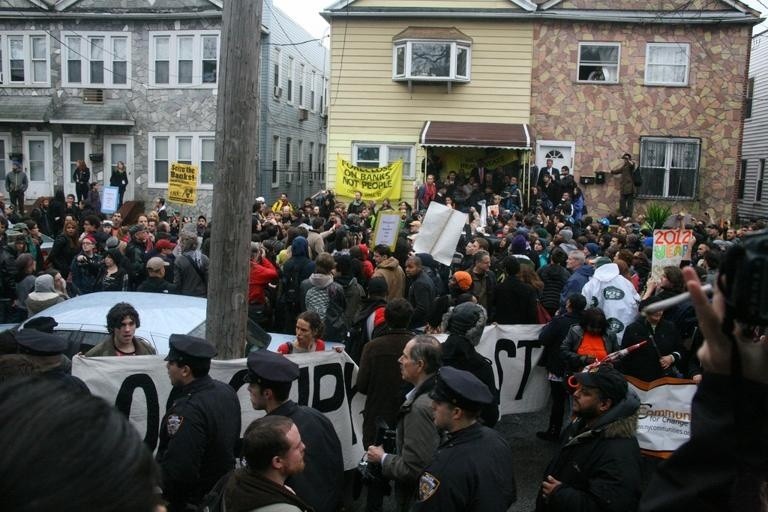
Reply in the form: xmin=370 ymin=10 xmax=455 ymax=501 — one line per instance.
xmin=717 ymin=232 xmax=768 ymax=328
xmin=349 ymin=222 xmax=365 ymax=234
xmin=352 ymin=425 xmax=398 ymax=501
xmin=77 ymin=261 xmax=90 ymax=268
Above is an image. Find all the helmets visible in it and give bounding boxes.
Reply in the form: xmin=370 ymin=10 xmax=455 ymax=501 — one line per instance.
xmin=453 ymin=271 xmax=473 ymax=290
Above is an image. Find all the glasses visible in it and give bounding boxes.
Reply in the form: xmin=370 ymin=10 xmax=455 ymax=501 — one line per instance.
xmin=81 ymin=241 xmax=92 ymax=245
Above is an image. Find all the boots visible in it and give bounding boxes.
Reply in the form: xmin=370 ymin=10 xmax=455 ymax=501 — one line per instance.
xmin=536 ymin=425 xmax=560 ymax=442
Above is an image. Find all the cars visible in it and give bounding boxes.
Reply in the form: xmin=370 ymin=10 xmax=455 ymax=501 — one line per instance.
xmin=1 ymin=293 xmax=345 ymax=355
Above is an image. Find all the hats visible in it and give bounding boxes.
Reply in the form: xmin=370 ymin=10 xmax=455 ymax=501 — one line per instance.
xmin=104 ymin=236 xmax=123 ymax=252
xmin=146 ymin=256 xmax=170 ymax=271
xmin=572 ymin=360 xmax=629 ymax=400
xmin=427 ymin=365 xmax=495 ymax=413
xmin=440 ymin=298 xmax=488 ymax=348
xmin=584 ymin=241 xmax=601 ymax=259
xmin=596 ymin=217 xmax=610 ymax=227
xmin=12 ymin=325 xmax=69 ymax=358
xmin=476 ymin=227 xmax=489 ymax=238
xmin=510 ymin=235 xmax=527 ymax=254
xmin=155 ymin=238 xmax=176 ymax=249
xmin=164 ymin=332 xmax=220 ymax=366
xmin=243 ymin=349 xmax=302 ymax=387
xmin=559 ymin=229 xmax=573 ymax=244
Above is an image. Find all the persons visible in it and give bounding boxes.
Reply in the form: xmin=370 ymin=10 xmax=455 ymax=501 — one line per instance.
xmin=156 ymin=333 xmax=243 ymax=511
xmin=69 ymin=235 xmax=106 ymax=295
xmin=86 ymin=181 xmax=101 ymax=212
xmin=491 ymin=256 xmax=536 ymax=325
xmin=136 ymin=256 xmax=173 ymax=296
xmin=78 ymin=217 xmax=103 ymax=241
xmin=146 ymin=219 xmax=158 ymax=238
xmin=295 ymin=251 xmax=346 ymax=342
xmin=618 ymin=296 xmax=685 ymax=381
xmin=281 ymin=236 xmax=316 ymax=307
xmin=104 ymin=236 xmax=133 ymax=292
xmin=0 ymin=214 xmax=10 ymax=250
xmin=28 ymin=220 xmax=45 ymax=271
xmin=352 ymin=244 xmax=374 ymax=278
xmin=1 ymin=360 xmax=160 ymax=511
xmin=154 ymin=219 xmax=177 ymax=243
xmin=434 ymin=270 xmax=480 ymax=330
xmin=536 ymin=291 xmax=592 ymax=442
xmin=365 ymin=333 xmax=456 ymax=511
xmin=12 ymin=253 xmax=37 ymax=323
xmin=174 ymin=232 xmax=212 ymax=296
xmin=155 ymin=196 xmax=170 ymax=223
xmin=536 ymin=249 xmax=575 ymax=321
xmin=557 ymin=249 xmax=598 ymax=317
xmin=354 ymin=297 xmax=417 ymax=510
xmin=370 ymin=244 xmax=406 ymax=303
xmin=30 ymin=196 xmax=50 ymax=235
xmin=193 ymin=214 xmax=209 ymax=238
xmin=437 ymin=302 xmax=502 ymax=428
xmin=461 ymin=249 xmax=498 ymax=325
xmin=126 ymin=224 xmax=149 ymax=292
xmin=98 ymin=220 xmax=115 ymax=241
xmin=135 ymin=214 xmax=158 ymax=244
xmin=5 ymin=162 xmax=29 ymax=212
xmin=56 ymin=215 xmax=76 ymax=236
xmin=46 ymin=222 xmax=81 ymax=280
xmin=26 ymin=276 xmax=67 ymax=320
xmin=252 ymin=153 xmax=767 ymax=253
xmin=147 ymin=212 xmax=161 ymax=222
xmin=563 ymin=310 xmax=626 ymax=373
xmin=639 ymin=266 xmax=689 ymax=312
xmin=0 ymin=228 xmax=31 ymax=324
xmin=75 ymin=200 xmax=92 ymax=218
xmin=96 ymin=252 xmax=129 ymax=292
xmin=240 ymin=348 xmax=348 ymax=512
xmin=74 ymin=302 xmax=161 ymax=359
xmin=152 ymin=240 xmax=172 ymax=263
xmin=578 ymin=257 xmax=644 ymax=351
xmin=403 ymin=255 xmax=443 ymax=329
xmin=410 ymin=365 xmax=518 ymax=511
xmin=351 ymin=277 xmax=392 ymax=363
xmin=110 ymin=158 xmax=130 ymax=210
xmin=60 ymin=194 xmax=81 ymax=217
xmin=535 ymin=369 xmax=642 ymax=510
xmin=71 ymin=159 xmax=90 ymax=203
xmin=106 ymin=211 xmax=125 ymax=240
xmin=246 ymin=247 xmax=277 ymax=331
xmin=275 ymin=309 xmax=344 ymax=358
xmin=205 ymin=415 xmax=313 ymax=511
xmin=641 ymin=263 xmax=768 ymax=512
